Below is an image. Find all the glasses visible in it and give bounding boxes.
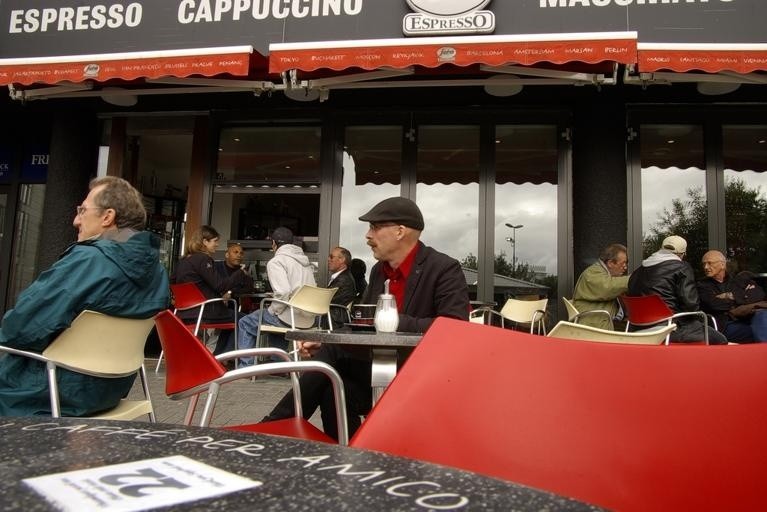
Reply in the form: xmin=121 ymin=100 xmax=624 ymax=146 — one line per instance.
xmin=75 ymin=205 xmax=103 ymax=214
xmin=328 ymin=253 xmax=340 ymax=260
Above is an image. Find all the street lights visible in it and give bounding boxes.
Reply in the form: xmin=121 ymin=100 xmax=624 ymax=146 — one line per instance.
xmin=506 ymin=222 xmax=522 ymax=277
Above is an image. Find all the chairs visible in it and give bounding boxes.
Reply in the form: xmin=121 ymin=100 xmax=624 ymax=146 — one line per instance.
xmin=467 ymin=296 xmax=763 ymax=345
xmin=342 ymin=315 xmax=767 ymax=512
xmin=3 ymin=311 xmax=158 ymax=423
xmin=153 ymin=282 xmax=377 ymax=379
xmin=153 ymin=309 xmax=349 ymax=446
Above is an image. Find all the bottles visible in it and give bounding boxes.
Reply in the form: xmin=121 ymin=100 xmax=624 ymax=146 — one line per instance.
xmin=374 ymin=293 xmax=401 ymax=334
xmin=252 ymin=280 xmax=262 ymax=291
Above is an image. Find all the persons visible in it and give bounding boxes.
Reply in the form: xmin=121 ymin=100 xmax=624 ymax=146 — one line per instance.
xmin=697 ymin=249 xmax=767 ymax=344
xmin=172 ymin=224 xmax=248 ymax=354
xmin=627 ymin=235 xmax=729 ymax=344
xmin=214 ymin=243 xmax=253 ymax=307
xmin=315 ymin=246 xmax=356 ymax=330
xmin=0 ymin=175 xmax=169 ymax=417
xmin=573 ymin=242 xmax=636 ymax=334
xmin=237 ymin=227 xmax=318 ymax=368
xmin=258 ymin=197 xmax=469 ymax=442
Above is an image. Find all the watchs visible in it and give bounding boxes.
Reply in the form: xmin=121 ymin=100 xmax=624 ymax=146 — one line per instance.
xmin=227 ymin=290 xmax=233 ymax=295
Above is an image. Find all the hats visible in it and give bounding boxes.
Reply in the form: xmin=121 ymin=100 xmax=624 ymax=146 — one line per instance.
xmin=661 ymin=235 xmax=688 ymax=256
xmin=358 ymin=196 xmax=425 ymax=231
xmin=271 ymin=226 xmax=294 ymax=244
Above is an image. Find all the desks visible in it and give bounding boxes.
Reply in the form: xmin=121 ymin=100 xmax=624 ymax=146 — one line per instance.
xmin=1 ymin=416 xmax=609 ymax=512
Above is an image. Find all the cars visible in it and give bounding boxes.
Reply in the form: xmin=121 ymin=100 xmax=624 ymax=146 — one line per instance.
xmin=751 ymin=272 xmax=767 ymax=292
